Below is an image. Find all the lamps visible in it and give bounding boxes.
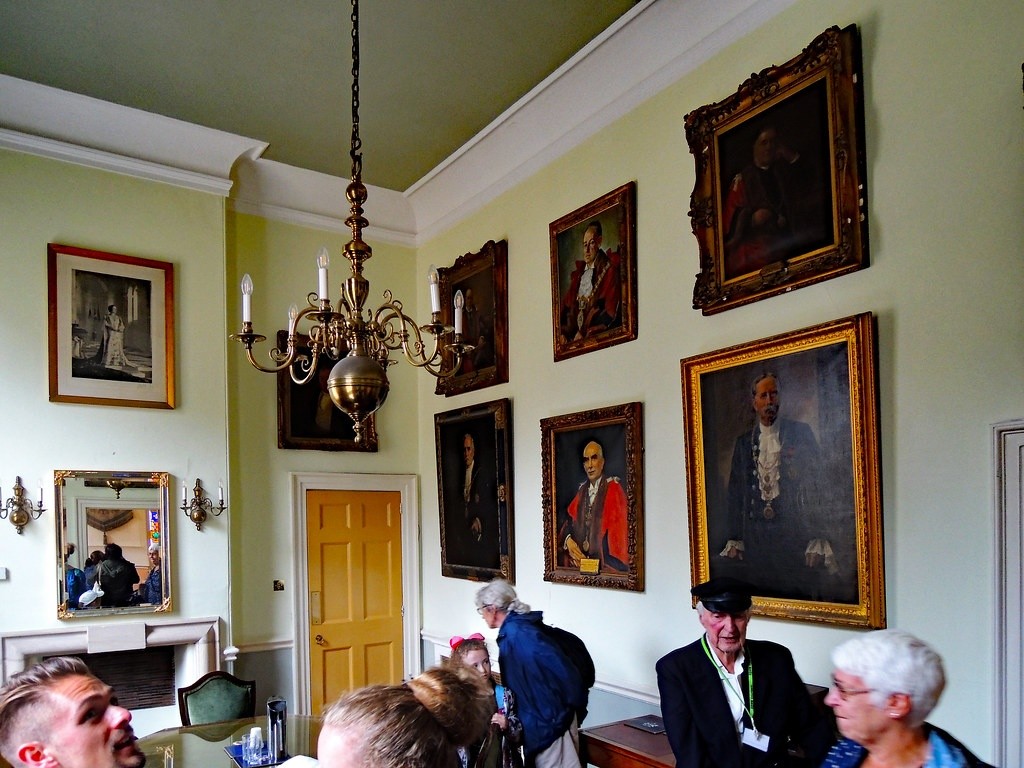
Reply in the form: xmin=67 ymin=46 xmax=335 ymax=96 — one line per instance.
xmin=230 ymin=0 xmax=475 ymax=443
xmin=180 ymin=478 xmax=228 ymax=531
xmin=0 ymin=476 xmax=46 ymax=534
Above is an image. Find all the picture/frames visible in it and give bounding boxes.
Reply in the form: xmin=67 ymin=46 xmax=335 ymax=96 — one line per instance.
xmin=277 ymin=330 xmax=378 ymax=452
xmin=684 ymin=24 xmax=870 ymax=316
xmin=540 ymin=402 xmax=645 ymax=593
xmin=434 ymin=239 xmax=509 ymax=398
xmin=549 ymin=181 xmax=638 ymax=362
xmin=680 ymin=311 xmax=887 ymax=629
xmin=433 ymin=398 xmax=516 ymax=586
xmin=47 ymin=243 xmax=175 ymax=410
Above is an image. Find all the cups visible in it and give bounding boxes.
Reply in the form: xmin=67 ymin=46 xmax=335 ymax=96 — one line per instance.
xmin=241 ymin=727 xmax=264 ymax=765
xmin=267 ymin=695 xmax=288 ymax=760
xmin=140 ymin=742 xmax=174 ymax=768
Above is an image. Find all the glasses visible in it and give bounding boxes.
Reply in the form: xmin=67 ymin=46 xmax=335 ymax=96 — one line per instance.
xmin=477 ymin=604 xmax=493 ymax=615
xmin=830 ymin=673 xmax=878 ymax=700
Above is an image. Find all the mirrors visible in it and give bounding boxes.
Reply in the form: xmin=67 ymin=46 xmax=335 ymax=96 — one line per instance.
xmin=53 ymin=470 xmax=173 ymax=620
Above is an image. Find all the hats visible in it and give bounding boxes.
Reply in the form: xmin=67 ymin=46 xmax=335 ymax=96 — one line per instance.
xmin=691 ymin=577 xmax=752 ymax=613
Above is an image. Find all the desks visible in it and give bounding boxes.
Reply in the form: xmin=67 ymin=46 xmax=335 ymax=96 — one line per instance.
xmin=577 ymin=714 xmax=676 ymax=768
xmin=136 ymin=716 xmax=323 ymax=768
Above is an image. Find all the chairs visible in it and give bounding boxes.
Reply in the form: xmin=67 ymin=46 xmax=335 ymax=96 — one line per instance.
xmin=178 ymin=671 xmax=256 ymax=726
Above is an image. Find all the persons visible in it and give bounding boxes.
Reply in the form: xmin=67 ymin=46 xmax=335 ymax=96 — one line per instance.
xmin=317 ymin=666 xmax=498 ymax=768
xmin=822 ymin=628 xmax=997 ymax=768
xmin=476 ymin=576 xmax=588 ymax=768
xmin=67 ymin=540 xmax=162 ymax=611
xmin=0 ymin=656 xmax=147 ymax=768
xmin=656 ymin=577 xmax=831 ymax=768
xmin=449 ymin=632 xmax=525 ymax=768
